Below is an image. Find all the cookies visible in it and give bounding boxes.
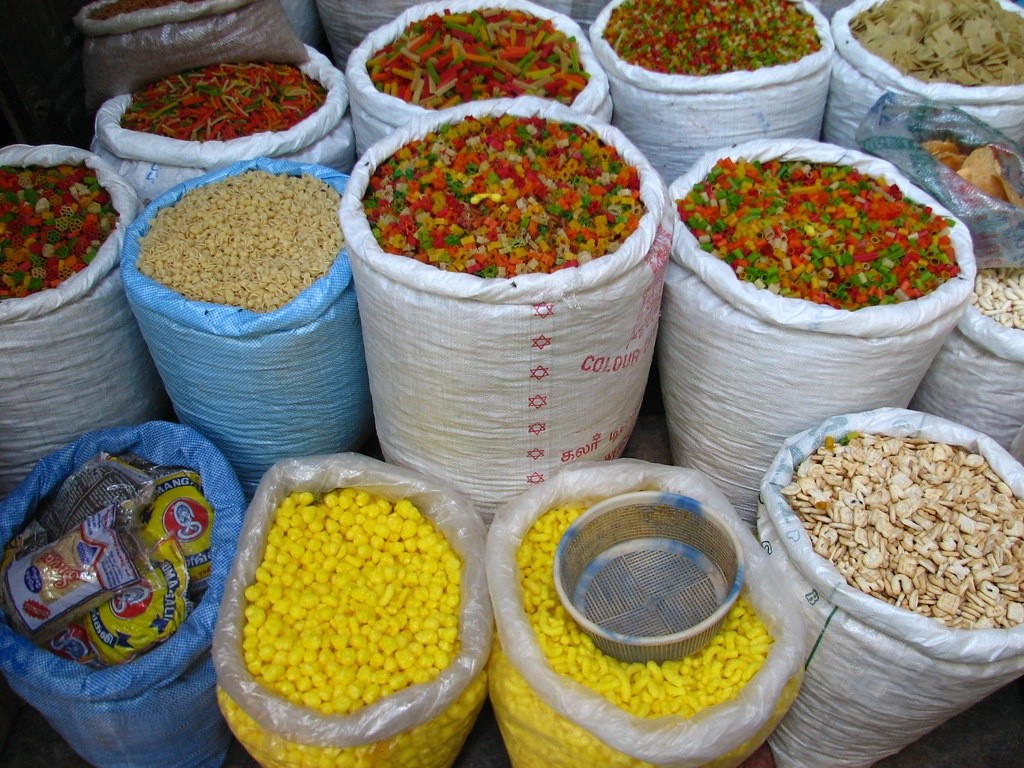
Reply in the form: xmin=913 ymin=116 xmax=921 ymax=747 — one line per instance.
xmin=781 ymin=436 xmax=1024 ymax=631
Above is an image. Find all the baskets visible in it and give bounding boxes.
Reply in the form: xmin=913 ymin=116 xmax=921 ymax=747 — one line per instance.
xmin=553 ymin=490 xmax=745 ymax=663
